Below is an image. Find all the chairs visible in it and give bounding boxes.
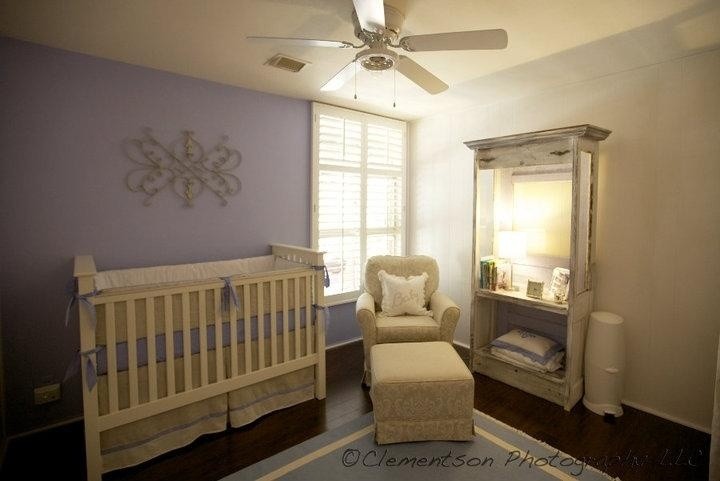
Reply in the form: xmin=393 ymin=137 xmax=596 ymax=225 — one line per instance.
xmin=354 ymin=254 xmax=461 ymax=387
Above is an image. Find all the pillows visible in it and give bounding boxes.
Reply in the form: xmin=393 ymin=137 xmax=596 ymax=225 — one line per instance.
xmin=489 ymin=327 xmax=563 ymax=364
xmin=377 ymin=270 xmax=434 ymax=318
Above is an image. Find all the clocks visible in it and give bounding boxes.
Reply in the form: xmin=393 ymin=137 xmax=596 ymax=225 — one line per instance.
xmin=526 ymin=279 xmax=544 ymax=300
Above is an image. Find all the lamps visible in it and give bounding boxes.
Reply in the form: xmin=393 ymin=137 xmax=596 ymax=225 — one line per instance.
xmin=495 ymin=231 xmax=525 ymax=291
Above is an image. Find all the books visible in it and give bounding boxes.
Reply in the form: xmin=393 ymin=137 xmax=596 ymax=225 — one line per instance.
xmin=480 ymin=255 xmax=512 ymax=291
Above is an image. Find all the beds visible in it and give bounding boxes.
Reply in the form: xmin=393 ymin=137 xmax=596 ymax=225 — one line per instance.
xmin=63 ymin=242 xmax=330 ymax=481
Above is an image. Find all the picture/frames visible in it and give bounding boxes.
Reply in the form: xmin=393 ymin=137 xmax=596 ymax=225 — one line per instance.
xmin=549 ymin=267 xmax=570 ymax=300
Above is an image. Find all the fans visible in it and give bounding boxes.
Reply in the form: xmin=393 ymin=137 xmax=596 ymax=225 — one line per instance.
xmin=247 ymin=0 xmax=508 ymax=103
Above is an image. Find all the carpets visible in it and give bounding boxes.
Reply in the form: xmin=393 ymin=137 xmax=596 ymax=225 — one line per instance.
xmin=217 ymin=408 xmax=619 ymax=481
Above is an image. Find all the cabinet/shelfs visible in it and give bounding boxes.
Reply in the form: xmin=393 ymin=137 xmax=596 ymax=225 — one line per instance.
xmin=463 ymin=123 xmax=612 ymax=411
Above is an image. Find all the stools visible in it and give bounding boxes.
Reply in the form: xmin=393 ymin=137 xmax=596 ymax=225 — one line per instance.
xmin=369 ymin=341 xmax=474 ymax=444
